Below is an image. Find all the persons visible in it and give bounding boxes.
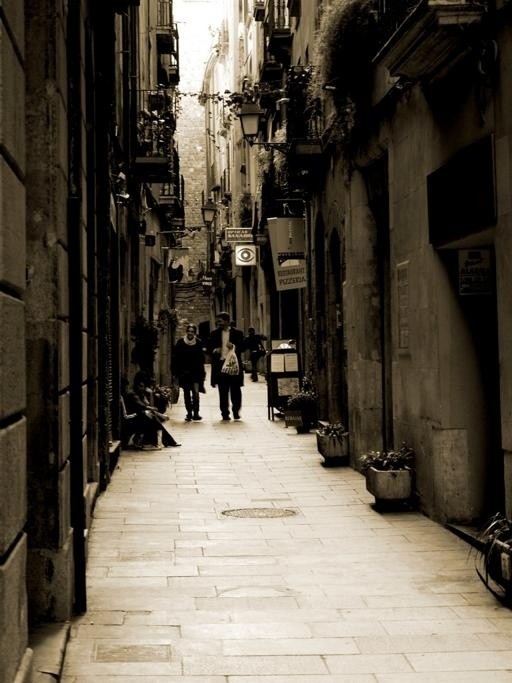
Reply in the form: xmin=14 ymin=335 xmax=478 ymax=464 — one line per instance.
xmin=243 ymin=328 xmax=268 ymax=382
xmin=117 ymin=367 xmax=169 ymax=451
xmin=209 ymin=312 xmax=243 ymax=421
xmin=230 ymin=320 xmax=237 ymax=327
xmin=171 ymin=324 xmax=206 ymax=421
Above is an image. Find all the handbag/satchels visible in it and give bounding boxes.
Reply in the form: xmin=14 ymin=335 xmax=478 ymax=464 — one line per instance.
xmin=222 ymin=345 xmax=240 ymax=375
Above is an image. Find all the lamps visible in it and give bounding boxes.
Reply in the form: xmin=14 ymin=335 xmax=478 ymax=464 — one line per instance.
xmin=237 ymin=95 xmax=291 ymax=155
xmin=183 ymin=197 xmax=218 ymax=230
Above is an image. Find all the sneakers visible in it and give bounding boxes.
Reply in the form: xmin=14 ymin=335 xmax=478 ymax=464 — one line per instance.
xmin=223 ymin=413 xmax=241 ymax=419
xmin=185 ymin=414 xmax=201 ymax=421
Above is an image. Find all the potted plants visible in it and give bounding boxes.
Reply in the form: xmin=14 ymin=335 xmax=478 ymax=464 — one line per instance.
xmin=358 ymin=441 xmax=418 ymax=500
xmin=466 ymin=511 xmax=512 ymax=591
xmin=285 ymin=392 xmax=318 ymax=435
xmin=316 ymin=423 xmax=349 ymax=457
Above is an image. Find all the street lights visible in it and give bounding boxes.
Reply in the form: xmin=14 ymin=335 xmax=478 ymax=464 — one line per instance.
xmin=203 ymin=196 xmax=217 ymax=274
